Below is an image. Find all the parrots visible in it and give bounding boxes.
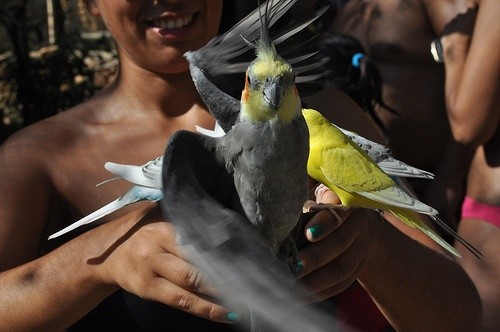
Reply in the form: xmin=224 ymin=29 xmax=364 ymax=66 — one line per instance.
xmin=42 ymin=0 xmax=364 ymax=332
xmin=300 ymin=106 xmax=486 ymax=262
xmin=317 ymin=32 xmax=405 ymax=147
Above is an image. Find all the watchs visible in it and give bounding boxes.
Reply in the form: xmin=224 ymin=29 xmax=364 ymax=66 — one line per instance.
xmin=430 ymin=19 xmax=474 ymax=63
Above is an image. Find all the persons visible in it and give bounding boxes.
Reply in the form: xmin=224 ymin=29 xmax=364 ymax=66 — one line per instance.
xmin=423 ymin=0 xmax=500 ymax=332
xmin=321 ymin=32 xmax=405 ymax=134
xmin=0 ymin=0 xmax=484 ymax=332
